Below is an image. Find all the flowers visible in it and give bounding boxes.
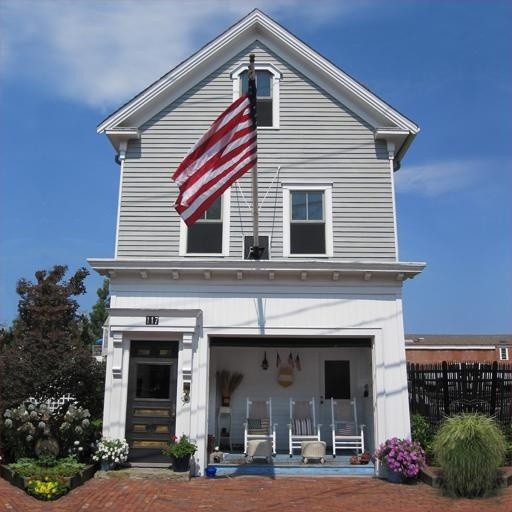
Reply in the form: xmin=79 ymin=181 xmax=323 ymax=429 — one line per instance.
xmin=161 ymin=434 xmax=198 ymax=459
xmin=374 ymin=437 xmax=425 ymax=478
xmin=90 ymin=439 xmax=129 ymax=463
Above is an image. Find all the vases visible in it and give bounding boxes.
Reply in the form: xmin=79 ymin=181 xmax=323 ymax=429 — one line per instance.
xmin=107 ymin=461 xmax=118 ymax=469
xmin=170 ymin=456 xmax=190 ymax=472
xmin=389 ymin=468 xmax=404 ymax=483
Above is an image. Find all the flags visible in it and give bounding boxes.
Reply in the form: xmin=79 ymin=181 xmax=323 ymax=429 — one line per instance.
xmin=168 ymin=91 xmax=257 ymax=229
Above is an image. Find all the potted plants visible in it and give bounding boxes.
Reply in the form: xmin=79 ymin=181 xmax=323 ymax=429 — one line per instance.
xmin=216 ymin=370 xmax=245 ymax=406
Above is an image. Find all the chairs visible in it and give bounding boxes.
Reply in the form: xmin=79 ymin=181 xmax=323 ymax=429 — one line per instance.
xmin=329 ymin=397 xmax=366 ymax=458
xmin=287 ymin=397 xmax=323 ymax=458
xmin=243 ymin=396 xmax=278 ymax=458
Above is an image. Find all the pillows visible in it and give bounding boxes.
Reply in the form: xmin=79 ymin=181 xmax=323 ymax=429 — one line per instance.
xmin=246 ymin=416 xmax=269 ymax=432
xmin=335 ymin=423 xmax=353 ymax=436
xmin=290 ymin=416 xmax=312 ymax=435
